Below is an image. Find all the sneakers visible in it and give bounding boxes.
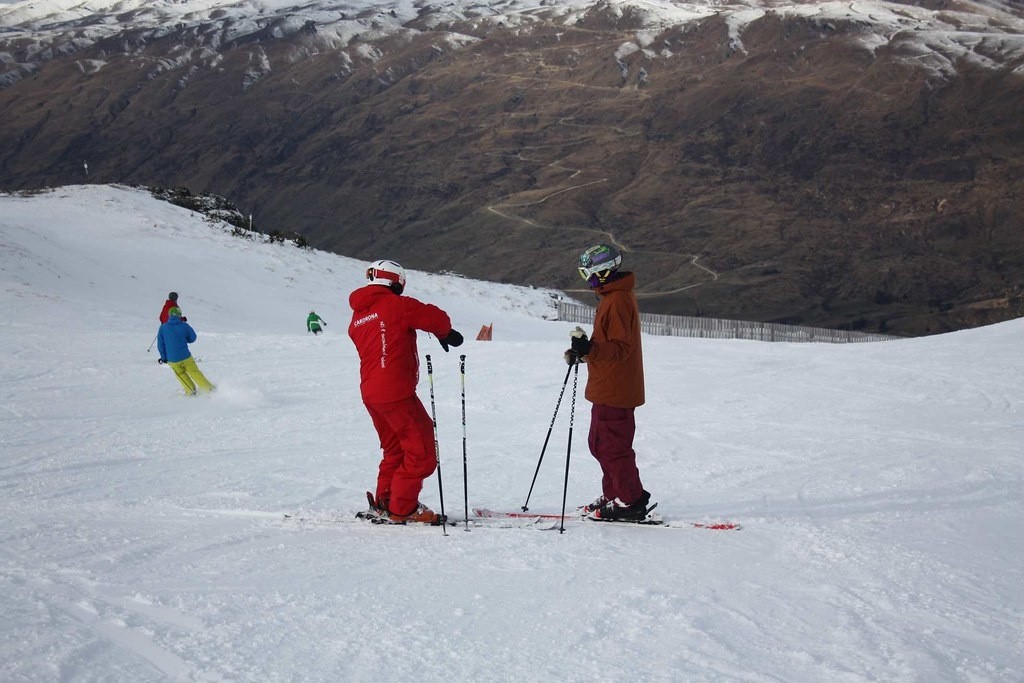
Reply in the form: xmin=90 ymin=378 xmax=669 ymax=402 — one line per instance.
xmin=388 ymin=500 xmax=447 ymax=524
xmin=582 ymin=491 xmax=653 ymax=523
xmin=366 ymin=491 xmax=388 ymax=516
xmin=583 ymin=494 xmax=610 ymax=513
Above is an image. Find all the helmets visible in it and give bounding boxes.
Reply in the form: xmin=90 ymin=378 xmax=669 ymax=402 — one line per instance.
xmin=167 ymin=306 xmax=182 ymax=316
xmin=308 ymin=309 xmax=315 ymax=314
xmin=169 ymin=292 xmax=178 ymax=299
xmin=577 ymin=240 xmax=624 ymax=272
xmin=365 ymin=259 xmax=407 ymax=294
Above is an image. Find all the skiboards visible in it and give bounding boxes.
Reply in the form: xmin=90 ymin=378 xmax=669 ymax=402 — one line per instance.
xmin=471 ymin=506 xmax=742 ymax=532
xmin=280 ymin=506 xmax=561 ymax=532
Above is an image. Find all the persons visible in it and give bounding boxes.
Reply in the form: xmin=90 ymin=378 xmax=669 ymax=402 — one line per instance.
xmin=346 ymin=258 xmax=464 ymax=525
xmin=160 ymin=292 xmax=187 ymax=323
xmin=564 ymin=244 xmax=651 ymax=520
xmin=157 ymin=306 xmax=214 ymax=396
xmin=307 ymin=310 xmax=327 ymax=335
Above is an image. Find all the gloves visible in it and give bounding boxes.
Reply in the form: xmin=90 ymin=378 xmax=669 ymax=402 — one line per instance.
xmin=324 ymin=323 xmax=327 ymax=325
xmin=180 ymin=316 xmax=187 ymax=322
xmin=438 ymin=329 xmax=464 ymax=353
xmin=563 ymin=348 xmax=588 ymax=365
xmin=569 ymin=326 xmax=591 ymax=357
xmin=158 ymin=359 xmax=168 ymax=364
xmin=307 ymin=328 xmax=311 ymax=332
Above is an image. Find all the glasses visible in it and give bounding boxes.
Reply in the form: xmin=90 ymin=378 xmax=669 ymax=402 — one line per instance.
xmin=577 ymin=254 xmax=621 ymax=282
